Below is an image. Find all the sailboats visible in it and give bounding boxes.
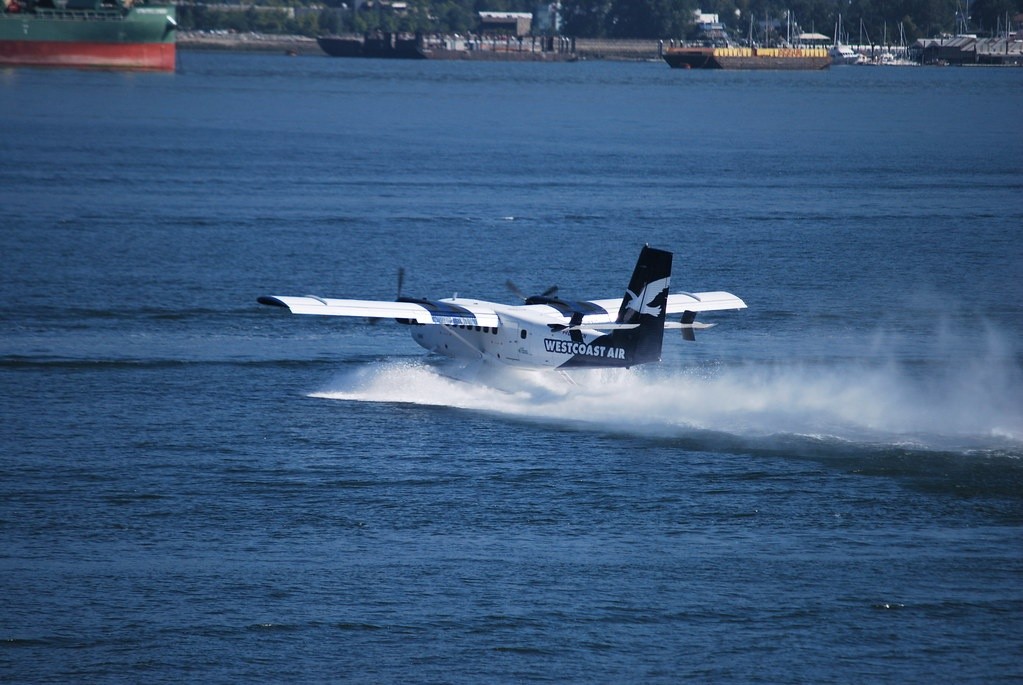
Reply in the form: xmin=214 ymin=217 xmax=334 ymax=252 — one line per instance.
xmin=828 ymin=14 xmax=919 ymax=67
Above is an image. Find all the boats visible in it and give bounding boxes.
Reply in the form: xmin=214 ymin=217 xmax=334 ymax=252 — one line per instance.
xmin=1 ymin=0 xmax=179 ymax=71
xmin=316 ymin=27 xmax=433 ymax=59
xmin=661 ymin=21 xmax=834 ymax=70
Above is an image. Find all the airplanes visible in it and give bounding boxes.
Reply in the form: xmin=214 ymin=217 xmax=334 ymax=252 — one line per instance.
xmin=255 ymin=247 xmax=749 ymax=401
